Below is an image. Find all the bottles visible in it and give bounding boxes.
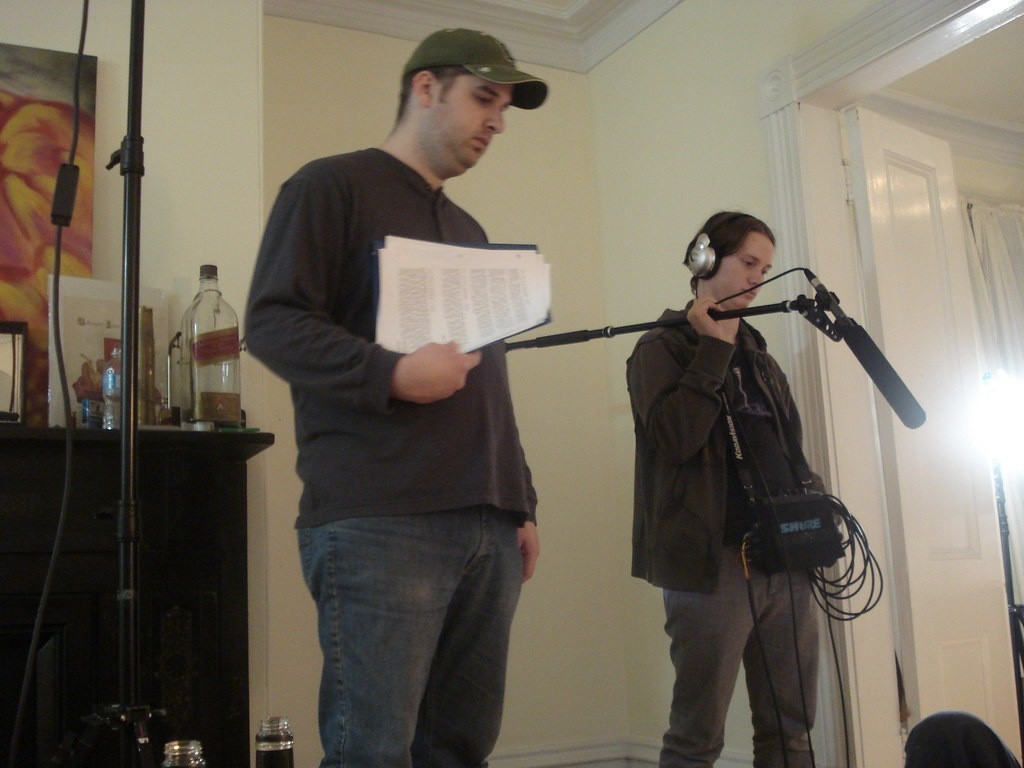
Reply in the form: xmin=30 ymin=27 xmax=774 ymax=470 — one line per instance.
xmin=162 ymin=740 xmax=205 ymax=768
xmin=255 ymin=717 xmax=294 ymax=768
xmin=180 ymin=264 xmax=241 ymax=429
xmin=102 ymin=346 xmax=124 ymax=430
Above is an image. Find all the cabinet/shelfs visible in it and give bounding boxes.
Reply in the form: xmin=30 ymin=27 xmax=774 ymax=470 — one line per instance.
xmin=0 ymin=425 xmax=275 ymax=768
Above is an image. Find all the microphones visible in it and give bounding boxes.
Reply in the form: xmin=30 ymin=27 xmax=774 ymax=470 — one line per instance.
xmin=803 ymin=268 xmax=926 ymax=430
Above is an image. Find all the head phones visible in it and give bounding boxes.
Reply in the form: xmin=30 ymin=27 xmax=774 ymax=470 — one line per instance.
xmin=687 ymin=212 xmax=752 ymax=280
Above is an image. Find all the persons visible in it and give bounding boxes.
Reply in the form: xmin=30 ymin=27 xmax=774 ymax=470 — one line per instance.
xmin=244 ymin=27 xmax=549 ymax=768
xmin=626 ymin=208 xmax=819 ymax=768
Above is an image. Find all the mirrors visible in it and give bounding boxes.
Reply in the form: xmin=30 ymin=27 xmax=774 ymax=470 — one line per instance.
xmin=0 ymin=320 xmax=29 ymax=425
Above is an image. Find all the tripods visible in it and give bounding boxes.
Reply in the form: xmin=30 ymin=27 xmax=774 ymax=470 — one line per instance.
xmin=44 ymin=0 xmax=177 ymax=768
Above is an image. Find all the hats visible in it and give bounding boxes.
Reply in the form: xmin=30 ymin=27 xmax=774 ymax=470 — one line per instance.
xmin=403 ymin=27 xmax=547 ymax=109
